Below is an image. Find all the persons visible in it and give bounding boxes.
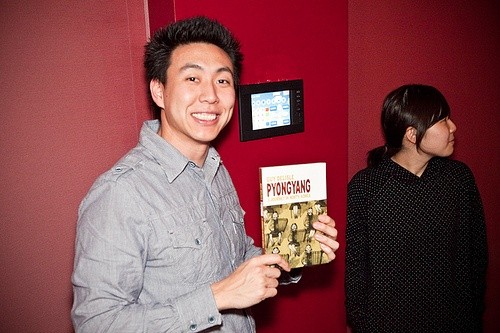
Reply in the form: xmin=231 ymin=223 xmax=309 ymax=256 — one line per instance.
xmin=69 ymin=14 xmax=339 ymax=333
xmin=345 ymin=84 xmax=487 ymax=333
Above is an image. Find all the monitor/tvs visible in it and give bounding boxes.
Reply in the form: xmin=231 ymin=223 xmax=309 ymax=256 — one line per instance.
xmin=238 ymin=79 xmax=304 ymax=141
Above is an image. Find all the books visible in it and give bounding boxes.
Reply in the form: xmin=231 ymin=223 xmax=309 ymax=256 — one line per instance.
xmin=259 ymin=162 xmax=333 ymax=269
